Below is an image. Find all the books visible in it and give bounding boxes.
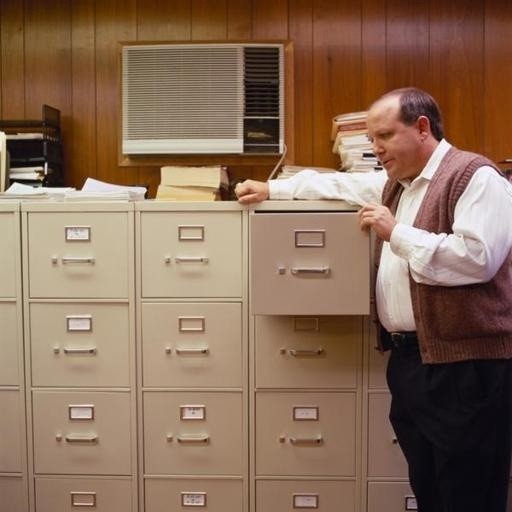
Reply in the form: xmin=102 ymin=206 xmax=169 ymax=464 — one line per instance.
xmin=330 ymin=109 xmax=384 ymax=174
xmin=159 ymin=164 xmax=228 ymax=189
xmin=155 ymin=183 xmax=225 ymax=202
xmin=66 ymin=173 xmax=148 ymax=202
xmin=0 ymin=130 xmax=76 ymax=198
xmin=277 ymin=162 xmax=336 ymax=181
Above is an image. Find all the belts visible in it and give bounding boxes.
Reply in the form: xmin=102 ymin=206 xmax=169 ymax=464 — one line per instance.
xmin=384 ymin=332 xmax=419 ymax=350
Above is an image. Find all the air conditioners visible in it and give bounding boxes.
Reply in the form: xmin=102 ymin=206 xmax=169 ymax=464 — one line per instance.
xmin=122 ymin=44 xmax=284 ymax=157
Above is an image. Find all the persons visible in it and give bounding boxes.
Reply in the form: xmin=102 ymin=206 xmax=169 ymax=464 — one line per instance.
xmin=235 ymin=86 xmax=512 ymax=512
